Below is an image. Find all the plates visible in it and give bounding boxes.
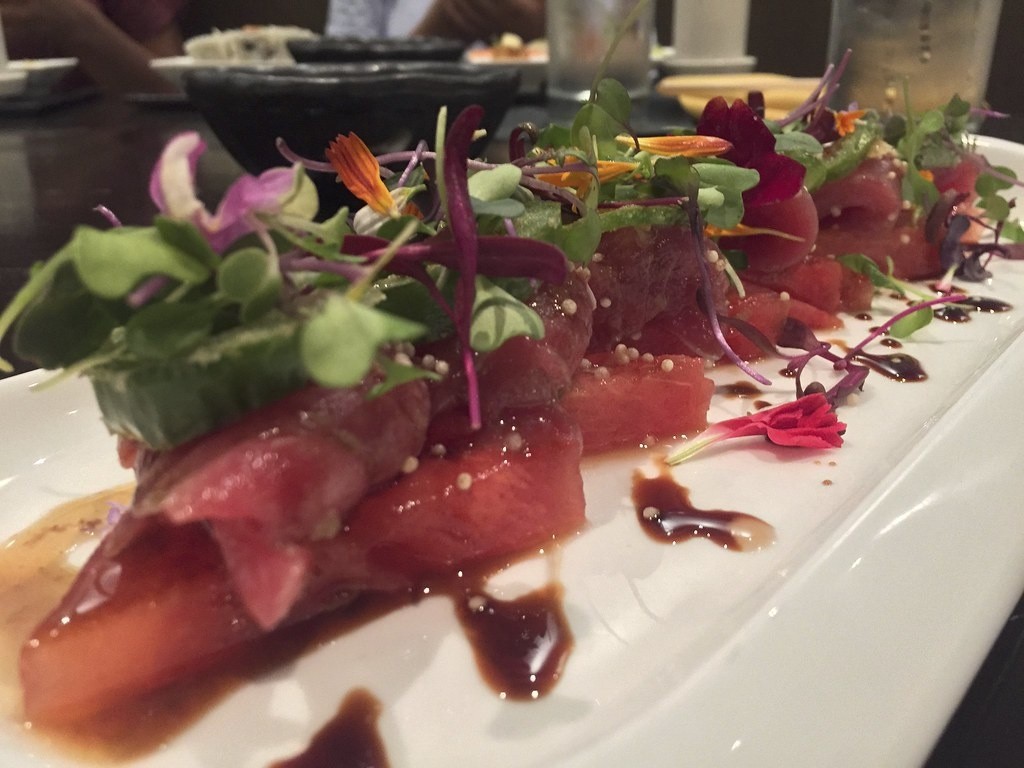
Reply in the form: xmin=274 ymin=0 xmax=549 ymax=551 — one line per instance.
xmin=0 ymin=133 xmax=1024 ymax=768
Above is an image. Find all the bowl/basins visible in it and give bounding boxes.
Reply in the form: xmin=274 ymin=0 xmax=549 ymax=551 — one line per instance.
xmin=467 ymin=46 xmax=670 ymax=101
xmin=281 ymin=38 xmax=470 ymax=62
xmin=661 ymin=56 xmax=758 ymax=74
xmin=656 ymin=72 xmax=827 ymax=124
xmin=181 ymin=64 xmax=514 ymax=212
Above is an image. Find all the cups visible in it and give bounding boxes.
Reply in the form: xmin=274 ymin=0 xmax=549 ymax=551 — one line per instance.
xmin=829 ymin=0 xmax=1000 ymax=139
xmin=543 ymin=0 xmax=652 ymax=96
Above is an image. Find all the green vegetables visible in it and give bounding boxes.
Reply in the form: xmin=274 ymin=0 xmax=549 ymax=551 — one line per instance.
xmin=1 ymin=5 xmax=1024 ymax=382
